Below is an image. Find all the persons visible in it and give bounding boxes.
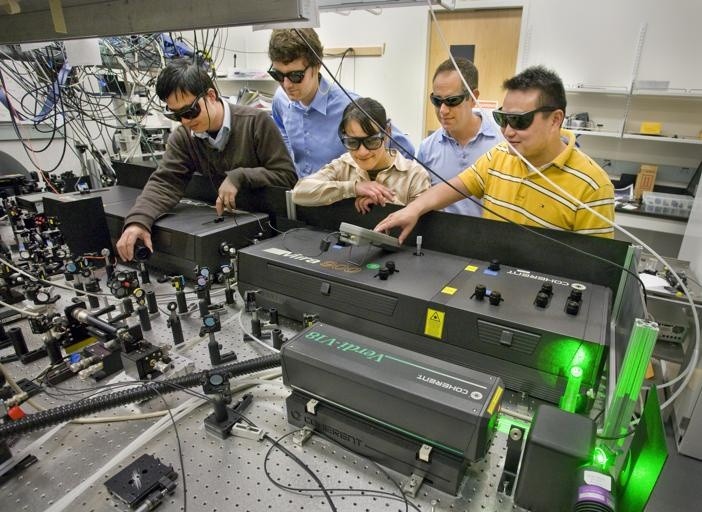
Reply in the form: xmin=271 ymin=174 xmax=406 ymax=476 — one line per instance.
xmin=417 ymin=57 xmax=507 ymax=218
xmin=290 ymin=97 xmax=432 ymax=215
xmin=114 ymin=58 xmax=299 ymax=263
xmin=265 ymin=30 xmax=415 ymax=178
xmin=372 ymin=63 xmax=615 ymax=245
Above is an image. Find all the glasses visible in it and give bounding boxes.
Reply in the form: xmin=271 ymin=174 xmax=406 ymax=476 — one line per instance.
xmin=339 ymin=134 xmax=386 ymax=151
xmin=160 ymin=92 xmax=209 ymax=122
xmin=429 ymin=91 xmax=471 ymax=107
xmin=490 ymin=106 xmax=559 ymax=131
xmin=266 ymin=63 xmax=314 ymax=83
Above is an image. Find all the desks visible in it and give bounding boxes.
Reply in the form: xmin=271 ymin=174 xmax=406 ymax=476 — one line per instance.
xmin=610 ymin=211 xmax=686 ymax=236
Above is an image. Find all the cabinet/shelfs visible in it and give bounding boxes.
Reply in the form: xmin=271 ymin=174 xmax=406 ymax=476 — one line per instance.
xmin=562 ymin=83 xmax=702 ymax=146
